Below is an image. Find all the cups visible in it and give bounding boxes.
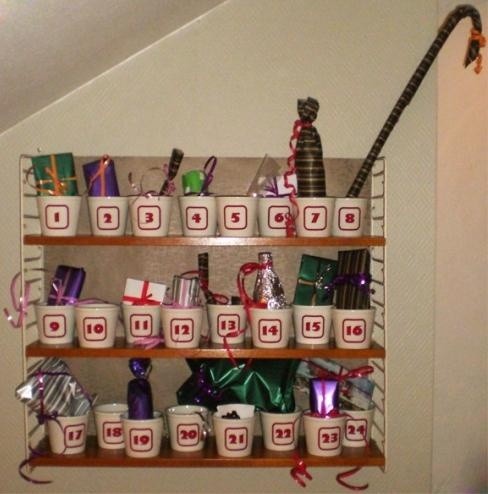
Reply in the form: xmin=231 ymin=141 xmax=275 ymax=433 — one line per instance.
xmin=33 ymin=300 xmax=378 ymax=351
xmin=35 ymin=195 xmax=369 ymax=239
xmin=46 ymin=402 xmax=376 ymax=460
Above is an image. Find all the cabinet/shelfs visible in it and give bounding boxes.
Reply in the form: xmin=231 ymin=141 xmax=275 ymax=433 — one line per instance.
xmin=18 ymin=235 xmax=386 ymax=469
